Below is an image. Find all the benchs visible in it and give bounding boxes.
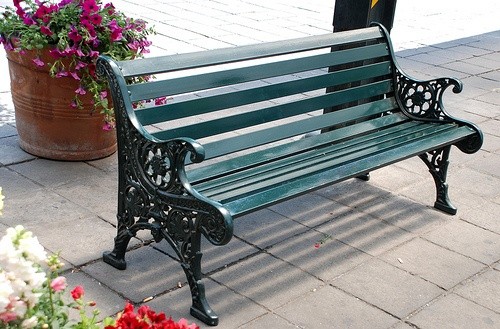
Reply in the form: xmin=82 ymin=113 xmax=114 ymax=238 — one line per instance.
xmin=96 ymin=22 xmax=484 ymax=327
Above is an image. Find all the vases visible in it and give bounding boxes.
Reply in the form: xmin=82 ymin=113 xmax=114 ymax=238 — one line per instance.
xmin=4 ymin=46 xmax=118 ymax=161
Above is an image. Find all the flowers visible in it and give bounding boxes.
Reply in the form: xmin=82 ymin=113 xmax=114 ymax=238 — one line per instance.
xmin=0 ymin=185 xmax=202 ymax=329
xmin=0 ymin=2 xmax=168 ymax=130
